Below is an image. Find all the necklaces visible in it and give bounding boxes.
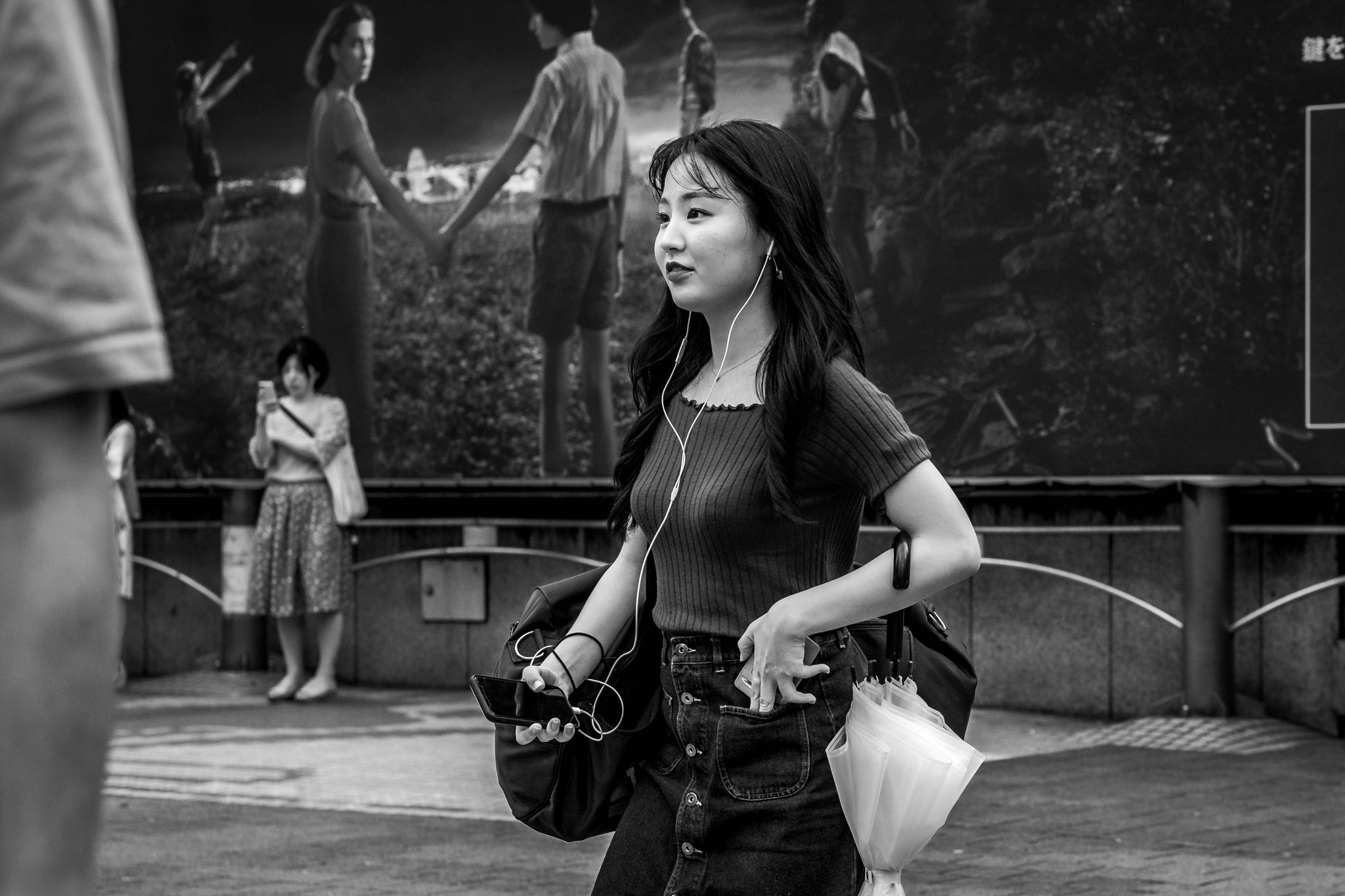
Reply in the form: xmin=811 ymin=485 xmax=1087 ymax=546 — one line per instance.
xmin=711 ymin=345 xmax=766 ymax=383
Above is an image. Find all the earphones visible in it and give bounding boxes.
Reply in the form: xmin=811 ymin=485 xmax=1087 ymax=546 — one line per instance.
xmin=767 ymin=239 xmax=775 ymax=255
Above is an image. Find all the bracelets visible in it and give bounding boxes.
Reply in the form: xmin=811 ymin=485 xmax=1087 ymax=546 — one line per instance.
xmin=554 ymin=631 xmax=604 ymax=673
xmin=550 ymin=648 xmax=576 ymax=692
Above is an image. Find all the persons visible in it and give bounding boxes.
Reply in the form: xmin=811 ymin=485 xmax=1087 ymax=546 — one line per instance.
xmin=248 ymin=334 xmax=350 ymax=705
xmin=513 ymin=122 xmax=984 ymax=896
xmin=679 ymin=0 xmax=720 ymax=141
xmin=1 ymin=0 xmax=176 ymax=895
xmin=179 ymin=47 xmax=255 ymax=262
xmin=303 ymin=5 xmax=440 ymax=475
xmin=815 ymin=30 xmax=882 ymax=331
xmin=93 ymin=389 xmax=141 ymax=693
xmin=430 ymin=0 xmax=630 ymax=477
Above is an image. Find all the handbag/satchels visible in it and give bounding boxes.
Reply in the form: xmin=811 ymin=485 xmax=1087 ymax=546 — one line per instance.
xmin=494 ymin=560 xmax=659 ymax=843
xmin=843 ymin=563 xmax=977 ymax=739
xmin=323 ymin=447 xmax=370 ymax=526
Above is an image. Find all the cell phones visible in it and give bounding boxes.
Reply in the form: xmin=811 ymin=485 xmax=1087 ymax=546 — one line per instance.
xmin=259 ymin=381 xmax=277 ymax=411
xmin=469 ymin=674 xmax=580 ymax=732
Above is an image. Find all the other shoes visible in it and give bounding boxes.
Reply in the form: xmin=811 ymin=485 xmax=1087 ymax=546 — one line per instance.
xmin=269 ymin=670 xmax=308 ymax=701
xmin=294 ymin=675 xmax=339 ymax=702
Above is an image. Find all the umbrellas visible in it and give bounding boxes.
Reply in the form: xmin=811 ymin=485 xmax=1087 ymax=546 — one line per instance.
xmin=822 ymin=530 xmax=982 ymax=895
